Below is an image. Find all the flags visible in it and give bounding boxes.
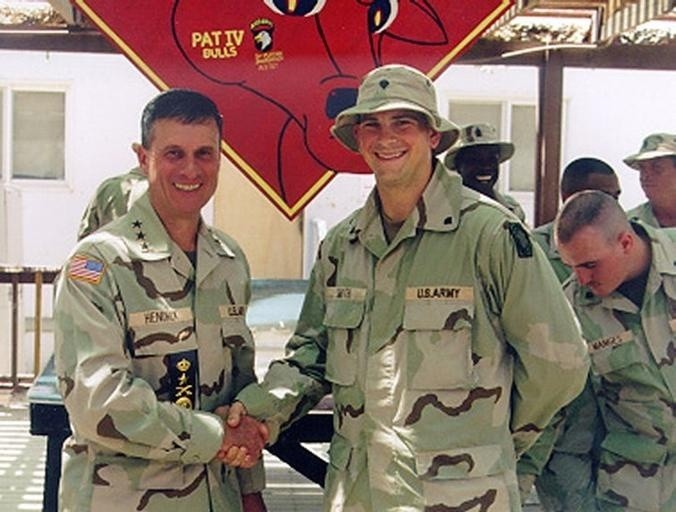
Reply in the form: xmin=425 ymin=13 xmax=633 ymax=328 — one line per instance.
xmin=69 ymin=256 xmax=103 ymax=281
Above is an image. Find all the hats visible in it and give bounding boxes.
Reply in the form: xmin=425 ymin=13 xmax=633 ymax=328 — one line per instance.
xmin=444 ymin=121 xmax=515 ymax=170
xmin=329 ymin=64 xmax=461 ymax=158
xmin=622 ymin=132 xmax=676 ymax=171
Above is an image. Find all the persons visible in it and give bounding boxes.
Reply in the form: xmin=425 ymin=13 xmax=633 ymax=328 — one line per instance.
xmin=514 ymin=190 xmax=676 ymax=512
xmin=445 ymin=124 xmax=526 ymax=222
xmin=531 ymin=157 xmax=622 ymax=512
xmin=623 ymin=132 xmax=676 ymax=230
xmin=78 ymin=167 xmax=149 ymax=241
xmin=53 ymin=88 xmax=266 ymax=512
xmin=219 ymin=63 xmax=590 ymax=512
